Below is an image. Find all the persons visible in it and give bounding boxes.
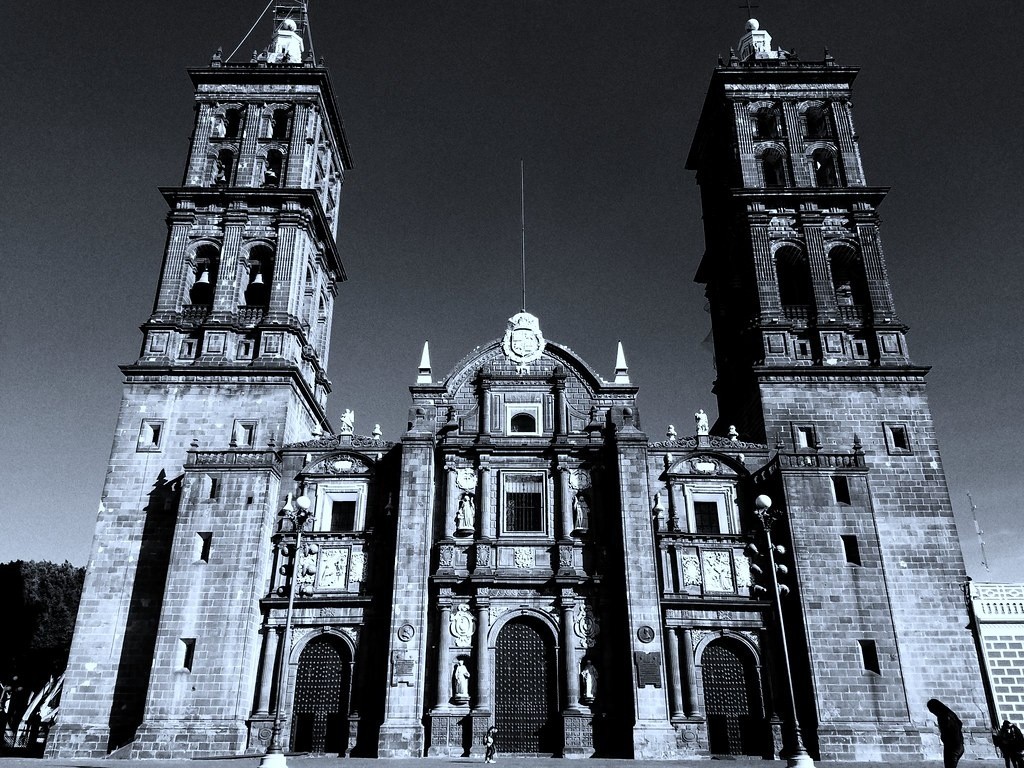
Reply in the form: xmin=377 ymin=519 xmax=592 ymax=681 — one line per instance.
xmin=927 ymin=699 xmax=965 ymax=768
xmin=574 ymin=496 xmax=589 ymax=527
xmin=459 ymin=496 xmax=475 ymax=527
xmin=454 ymin=660 xmax=470 ymax=694
xmin=340 ymin=408 xmax=352 ymax=427
xmin=485 ymin=726 xmax=498 ymax=764
xmin=997 ymin=720 xmax=1024 ymax=768
xmin=581 ymin=660 xmax=598 ymax=697
xmin=694 ymin=409 xmax=709 ymax=431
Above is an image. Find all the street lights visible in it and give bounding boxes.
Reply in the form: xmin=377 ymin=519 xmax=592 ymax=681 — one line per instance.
xmin=741 ymin=494 xmax=812 ymax=753
xmin=266 ymin=496 xmax=317 ymax=754
